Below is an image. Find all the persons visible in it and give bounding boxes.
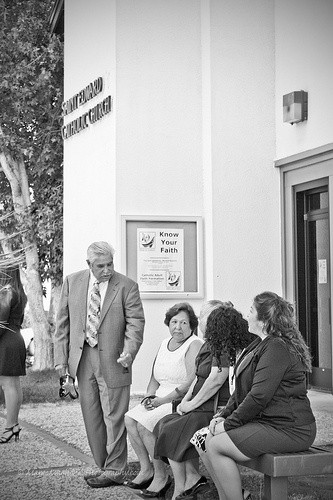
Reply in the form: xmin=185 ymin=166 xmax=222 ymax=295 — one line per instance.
xmin=124 ymin=302 xmax=205 ymax=498
xmin=53 ymin=243 xmax=145 ymax=488
xmin=0 ymin=259 xmax=28 ymax=444
xmin=205 ymin=291 xmax=316 ymax=500
xmin=152 ymin=299 xmax=262 ymax=500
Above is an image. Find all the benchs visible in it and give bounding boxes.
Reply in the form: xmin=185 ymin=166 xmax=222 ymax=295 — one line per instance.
xmin=239 ymin=446 xmax=333 ymax=500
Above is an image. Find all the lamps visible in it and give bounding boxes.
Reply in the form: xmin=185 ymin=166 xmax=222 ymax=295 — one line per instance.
xmin=282 ymin=91 xmax=306 ymax=124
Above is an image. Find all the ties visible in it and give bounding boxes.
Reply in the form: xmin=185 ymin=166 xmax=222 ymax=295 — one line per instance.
xmin=85 ymin=279 xmax=101 ymax=348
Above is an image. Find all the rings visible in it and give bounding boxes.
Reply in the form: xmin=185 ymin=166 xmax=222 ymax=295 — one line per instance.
xmin=215 ymin=421 xmax=218 ymax=423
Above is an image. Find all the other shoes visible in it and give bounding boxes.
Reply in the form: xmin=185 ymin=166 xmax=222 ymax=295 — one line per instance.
xmin=175 ymin=475 xmax=207 ymax=499
xmin=242 ymin=488 xmax=252 ymax=500
xmin=84 ymin=471 xmax=124 ymax=487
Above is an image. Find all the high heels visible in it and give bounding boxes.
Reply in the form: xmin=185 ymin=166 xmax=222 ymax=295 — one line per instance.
xmin=123 ymin=474 xmax=154 ymax=489
xmin=141 ymin=474 xmax=172 ymax=496
xmin=0 ymin=423 xmax=22 ymax=443
